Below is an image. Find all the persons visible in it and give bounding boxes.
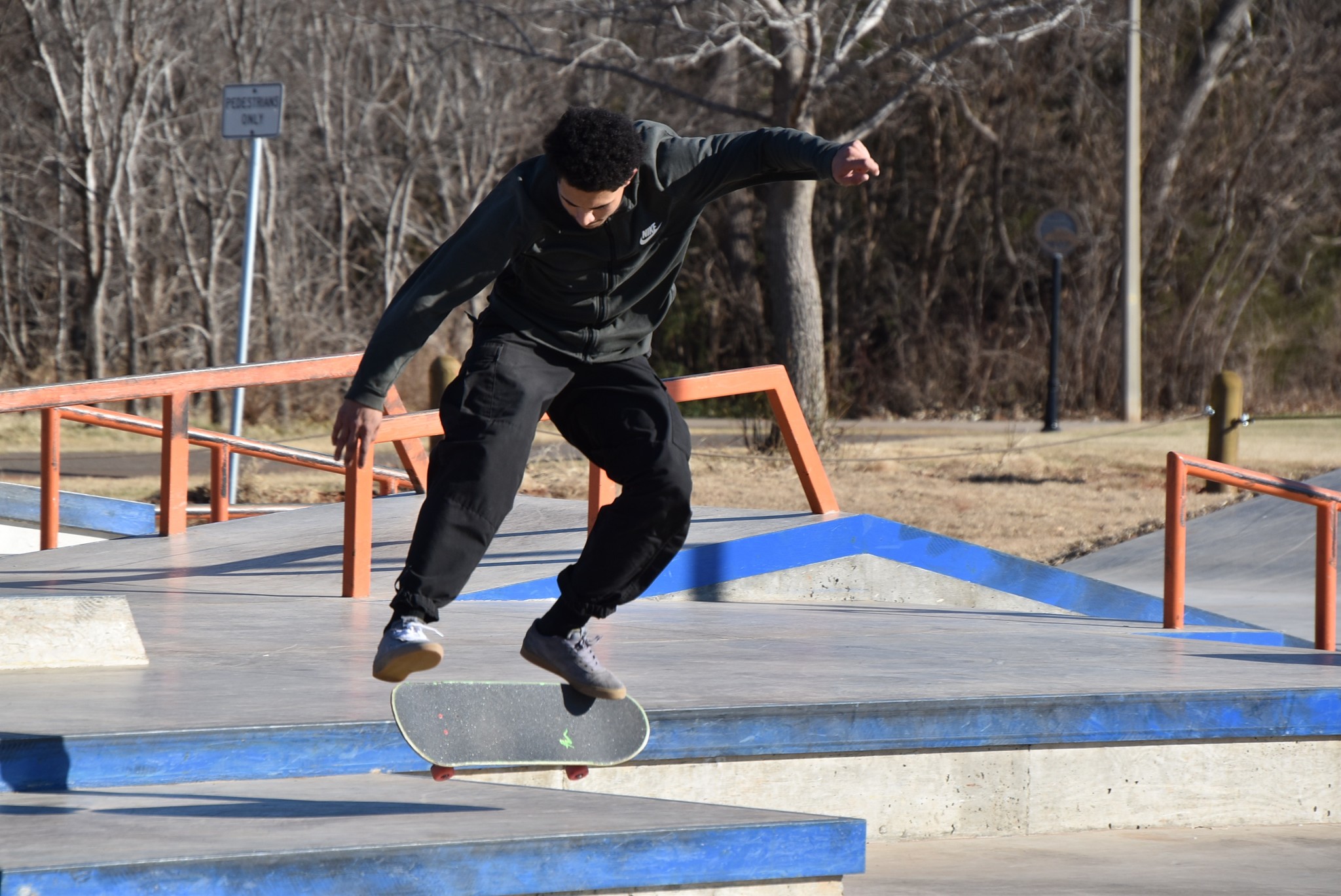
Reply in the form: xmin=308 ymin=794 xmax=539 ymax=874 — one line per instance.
xmin=330 ymin=107 xmax=880 ymax=700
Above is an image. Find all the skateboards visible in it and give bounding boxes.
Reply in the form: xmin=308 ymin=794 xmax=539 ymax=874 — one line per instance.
xmin=389 ymin=680 xmax=652 ymax=782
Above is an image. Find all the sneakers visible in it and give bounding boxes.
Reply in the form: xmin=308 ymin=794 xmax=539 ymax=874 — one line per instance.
xmin=520 ymin=618 xmax=626 ymax=699
xmin=373 ymin=617 xmax=443 ymax=682
